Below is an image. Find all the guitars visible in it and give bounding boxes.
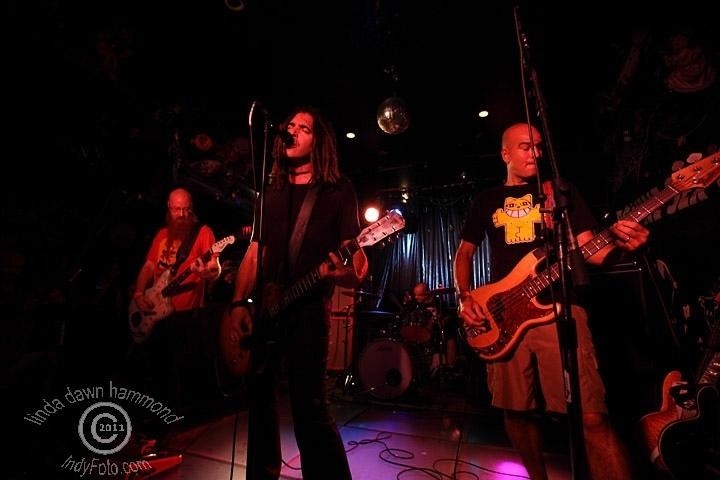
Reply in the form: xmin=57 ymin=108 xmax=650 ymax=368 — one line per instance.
xmin=464 ymin=151 xmax=718 ymax=360
xmin=639 ymin=354 xmax=720 ymax=478
xmin=128 ymin=235 xmax=236 ymax=343
xmin=217 ymin=209 xmax=407 ymax=376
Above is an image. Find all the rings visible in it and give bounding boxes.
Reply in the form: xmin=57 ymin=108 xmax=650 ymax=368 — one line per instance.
xmin=623 ymin=237 xmax=630 ymax=242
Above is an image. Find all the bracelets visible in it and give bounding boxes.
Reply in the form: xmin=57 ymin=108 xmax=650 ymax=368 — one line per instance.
xmin=226 ymin=297 xmax=255 ymax=313
xmin=456 ymin=289 xmax=470 ymax=301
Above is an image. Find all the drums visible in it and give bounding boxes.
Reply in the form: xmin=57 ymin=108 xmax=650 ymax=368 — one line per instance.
xmin=358 ymin=326 xmax=469 ymax=399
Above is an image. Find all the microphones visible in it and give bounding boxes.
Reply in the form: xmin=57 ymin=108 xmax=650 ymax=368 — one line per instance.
xmin=564 ymin=218 xmax=592 ymax=294
xmin=255 ymin=100 xmax=293 ymax=146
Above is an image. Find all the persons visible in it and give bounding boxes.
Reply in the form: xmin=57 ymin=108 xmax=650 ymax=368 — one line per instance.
xmin=452 ymin=123 xmax=650 ymax=480
xmin=224 ymin=108 xmax=369 ymax=480
xmin=413 ymin=283 xmax=433 ymax=303
xmin=134 ymin=189 xmax=222 ymax=456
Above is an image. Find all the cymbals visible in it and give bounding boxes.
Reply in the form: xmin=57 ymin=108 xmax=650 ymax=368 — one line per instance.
xmin=366 ymin=311 xmax=395 ymax=317
xmin=355 ymin=291 xmax=381 ymax=298
xmin=428 ymin=288 xmax=456 ymax=295
